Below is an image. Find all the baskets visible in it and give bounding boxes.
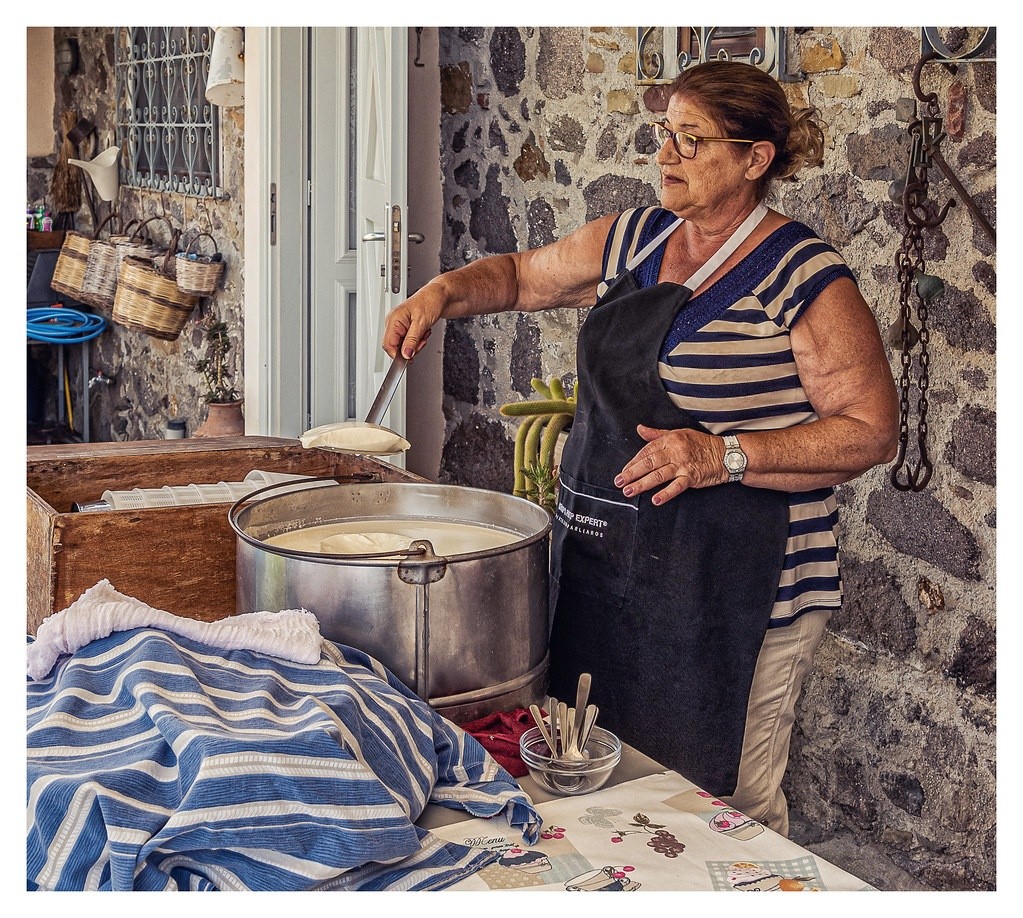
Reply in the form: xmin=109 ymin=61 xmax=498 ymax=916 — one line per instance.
xmin=50 ymin=211 xmax=222 ymax=342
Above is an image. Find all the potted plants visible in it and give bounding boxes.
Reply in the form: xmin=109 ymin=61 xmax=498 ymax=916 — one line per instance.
xmin=194 ymin=322 xmax=246 ymax=438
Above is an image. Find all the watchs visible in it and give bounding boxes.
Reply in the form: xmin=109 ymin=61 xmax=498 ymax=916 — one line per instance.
xmin=721 ymin=435 xmax=748 ymax=482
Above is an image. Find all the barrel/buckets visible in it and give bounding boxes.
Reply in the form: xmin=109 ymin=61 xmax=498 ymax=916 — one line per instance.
xmin=227 ymin=469 xmax=555 ymax=707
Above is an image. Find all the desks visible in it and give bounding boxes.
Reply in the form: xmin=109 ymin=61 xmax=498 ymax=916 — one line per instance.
xmin=422 ymin=693 xmax=881 ymax=891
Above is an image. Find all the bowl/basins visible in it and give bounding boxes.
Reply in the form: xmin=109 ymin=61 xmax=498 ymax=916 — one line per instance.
xmin=519 ymin=725 xmax=621 ymax=796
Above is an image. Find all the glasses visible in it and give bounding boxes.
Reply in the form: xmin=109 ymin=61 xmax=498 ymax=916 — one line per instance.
xmin=649 ymin=122 xmax=756 ymax=159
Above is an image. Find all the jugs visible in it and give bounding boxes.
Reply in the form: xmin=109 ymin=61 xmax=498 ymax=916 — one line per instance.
xmin=67 ymin=146 xmax=119 ymax=201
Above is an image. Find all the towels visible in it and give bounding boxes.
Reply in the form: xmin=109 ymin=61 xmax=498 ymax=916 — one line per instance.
xmin=26 ymin=577 xmax=325 ymax=682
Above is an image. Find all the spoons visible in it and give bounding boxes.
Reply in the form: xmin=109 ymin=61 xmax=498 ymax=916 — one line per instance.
xmin=529 ymin=673 xmax=599 ymax=793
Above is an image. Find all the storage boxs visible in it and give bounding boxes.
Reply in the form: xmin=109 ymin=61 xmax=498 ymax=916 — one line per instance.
xmin=28 ymin=449 xmax=444 ymax=636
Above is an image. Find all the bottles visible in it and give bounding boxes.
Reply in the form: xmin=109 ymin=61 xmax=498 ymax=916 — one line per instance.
xmin=27 ymin=203 xmax=53 ymax=233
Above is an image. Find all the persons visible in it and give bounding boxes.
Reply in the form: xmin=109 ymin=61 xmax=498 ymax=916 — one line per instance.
xmin=380 ymin=60 xmax=899 ymax=840
